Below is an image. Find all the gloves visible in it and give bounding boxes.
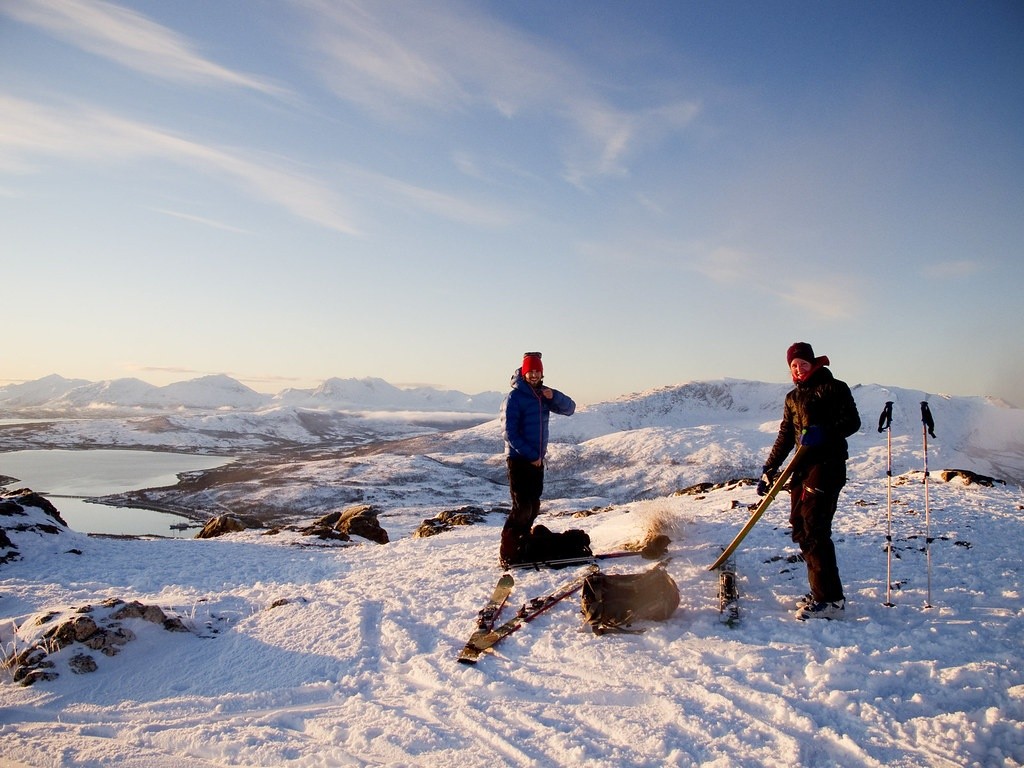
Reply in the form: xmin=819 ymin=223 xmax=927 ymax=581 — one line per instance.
xmin=800 ymin=424 xmax=822 ymax=446
xmin=757 ymin=465 xmax=777 ymax=496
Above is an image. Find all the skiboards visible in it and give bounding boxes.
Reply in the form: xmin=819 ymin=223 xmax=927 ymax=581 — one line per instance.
xmin=709 ymin=440 xmax=805 ymax=626
xmin=457 ymin=562 xmax=601 ymax=667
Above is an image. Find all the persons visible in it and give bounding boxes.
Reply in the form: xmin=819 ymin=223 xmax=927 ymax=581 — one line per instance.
xmin=499 ymin=351 xmax=576 ymax=566
xmin=757 ymin=343 xmax=860 ymax=621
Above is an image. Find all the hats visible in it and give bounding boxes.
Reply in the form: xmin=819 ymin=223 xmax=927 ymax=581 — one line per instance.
xmin=522 ymin=352 xmax=543 ymax=376
xmin=787 ymin=341 xmax=814 ymax=367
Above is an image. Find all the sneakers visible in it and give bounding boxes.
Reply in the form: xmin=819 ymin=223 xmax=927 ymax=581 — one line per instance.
xmin=794 ymin=590 xmax=846 ymax=621
